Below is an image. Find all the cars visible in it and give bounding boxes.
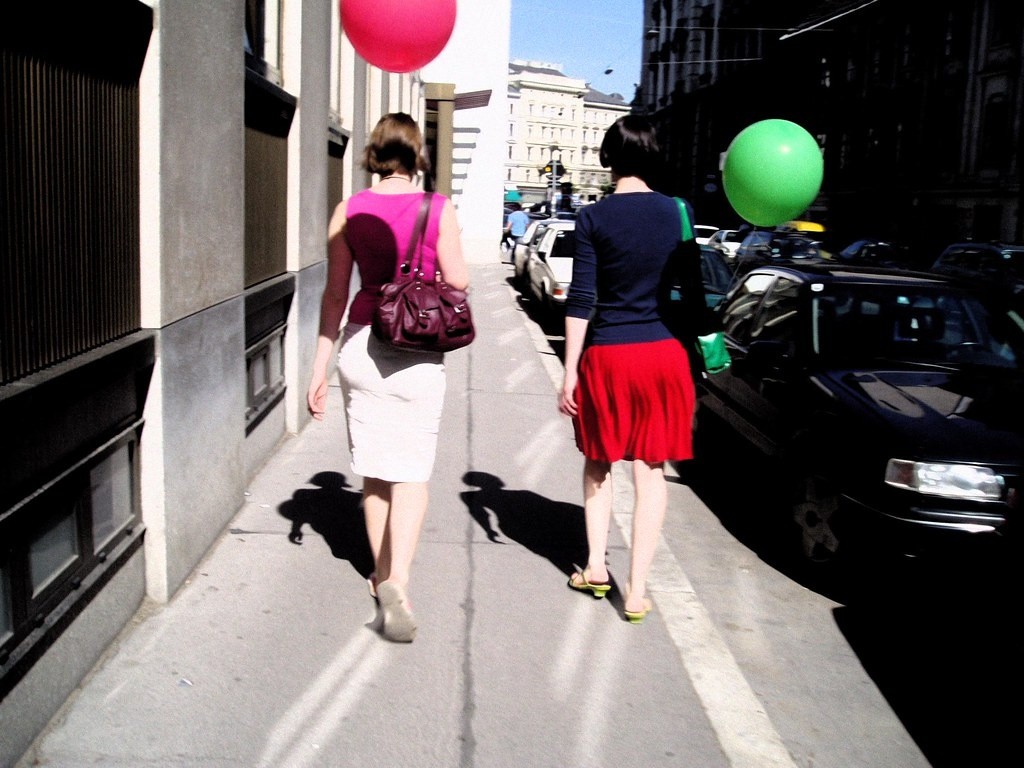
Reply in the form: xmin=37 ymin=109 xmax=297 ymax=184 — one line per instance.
xmin=686 ymin=261 xmax=1024 ymax=607
xmin=516 ymin=219 xmax=1024 ymax=346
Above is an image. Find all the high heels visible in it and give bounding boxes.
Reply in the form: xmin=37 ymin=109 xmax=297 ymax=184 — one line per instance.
xmin=568 ymin=571 xmax=611 ymax=597
xmin=626 ymin=598 xmax=654 ymax=625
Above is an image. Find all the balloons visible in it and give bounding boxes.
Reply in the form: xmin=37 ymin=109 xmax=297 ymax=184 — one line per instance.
xmin=339 ymin=0 xmax=456 ymax=74
xmin=723 ymin=120 xmax=824 ymax=227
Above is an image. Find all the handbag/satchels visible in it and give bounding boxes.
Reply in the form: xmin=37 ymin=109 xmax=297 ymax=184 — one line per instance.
xmin=675 ymin=196 xmax=732 ymax=375
xmin=372 ymin=192 xmax=475 ymax=353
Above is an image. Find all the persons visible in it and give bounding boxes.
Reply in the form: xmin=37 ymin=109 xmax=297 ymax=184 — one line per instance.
xmin=556 ymin=116 xmax=702 ymax=624
xmin=504 ymin=201 xmax=531 ymax=248
xmin=306 ymin=112 xmax=469 ymax=643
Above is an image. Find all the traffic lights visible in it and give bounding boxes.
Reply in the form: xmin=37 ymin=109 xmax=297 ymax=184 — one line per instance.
xmin=545 ymin=166 xmax=551 ymax=172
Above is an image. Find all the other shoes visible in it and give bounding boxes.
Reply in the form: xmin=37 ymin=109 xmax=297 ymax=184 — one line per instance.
xmin=367 ymin=569 xmax=388 ymax=598
xmin=376 ymin=580 xmax=419 ymax=644
